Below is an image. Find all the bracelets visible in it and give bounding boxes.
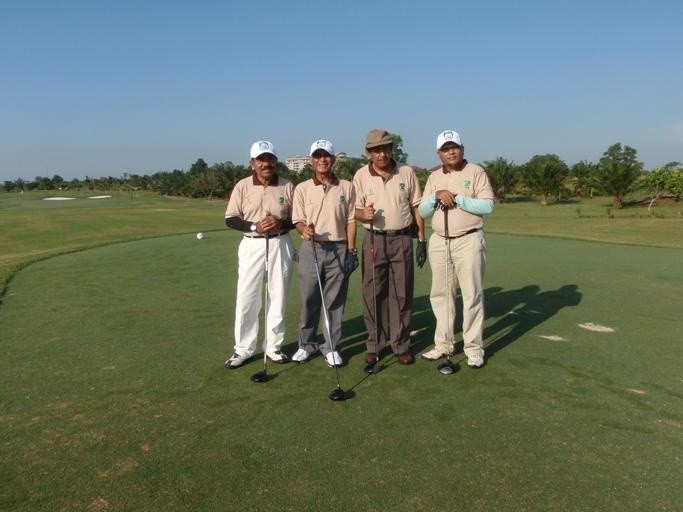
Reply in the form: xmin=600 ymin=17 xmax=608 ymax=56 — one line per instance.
xmin=418 ymin=240 xmax=426 ymax=243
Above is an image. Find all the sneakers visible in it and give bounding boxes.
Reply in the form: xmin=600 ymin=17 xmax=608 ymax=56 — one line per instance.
xmin=465 ymin=347 xmax=485 ymax=367
xmin=320 ymin=344 xmax=342 ymax=367
xmin=224 ymin=352 xmax=252 ymax=367
xmin=292 ymin=348 xmax=317 ymax=362
xmin=366 ymin=350 xmax=383 ymax=363
xmin=264 ymin=346 xmax=289 ymax=362
xmin=422 ymin=346 xmax=456 ymax=360
xmin=398 ymin=348 xmax=414 ymax=365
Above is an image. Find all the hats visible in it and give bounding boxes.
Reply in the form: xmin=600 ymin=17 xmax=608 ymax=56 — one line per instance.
xmin=436 ymin=130 xmax=464 ymax=148
xmin=310 ymin=140 xmax=336 ymax=157
xmin=365 ymin=129 xmax=394 ymax=148
xmin=250 ymin=140 xmax=279 ymax=160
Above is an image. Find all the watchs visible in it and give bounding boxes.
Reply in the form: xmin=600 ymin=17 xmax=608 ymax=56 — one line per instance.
xmin=348 ymin=248 xmax=357 ymax=253
xmin=250 ymin=222 xmax=257 ymax=232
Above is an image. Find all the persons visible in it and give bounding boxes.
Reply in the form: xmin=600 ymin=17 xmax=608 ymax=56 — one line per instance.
xmin=351 ymin=128 xmax=427 ymax=365
xmin=418 ymin=130 xmax=495 ymax=369
xmin=224 ymin=141 xmax=296 ymax=366
xmin=292 ymin=140 xmax=358 ymax=365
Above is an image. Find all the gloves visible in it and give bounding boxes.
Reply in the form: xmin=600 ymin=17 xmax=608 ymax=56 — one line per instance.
xmin=344 ymin=249 xmax=360 ymax=276
xmin=415 ymin=241 xmax=427 ymax=268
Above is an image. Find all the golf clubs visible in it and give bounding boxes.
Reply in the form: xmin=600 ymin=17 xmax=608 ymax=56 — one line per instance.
xmin=364 ymin=204 xmax=380 ymax=374
xmin=307 ymin=222 xmax=344 ymax=400
xmin=437 ymin=206 xmax=456 ymax=375
xmin=250 ymin=210 xmax=271 ymax=382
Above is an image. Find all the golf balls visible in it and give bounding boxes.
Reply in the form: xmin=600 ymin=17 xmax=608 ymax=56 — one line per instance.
xmin=196 ymin=232 xmax=203 ymax=239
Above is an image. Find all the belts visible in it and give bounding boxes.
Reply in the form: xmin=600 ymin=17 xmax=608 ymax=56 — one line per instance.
xmin=368 ymin=227 xmax=411 ymax=236
xmin=245 ymin=232 xmax=289 ymax=238
xmin=315 ymin=240 xmax=347 ymax=244
xmin=447 ymin=228 xmax=478 ymax=240
